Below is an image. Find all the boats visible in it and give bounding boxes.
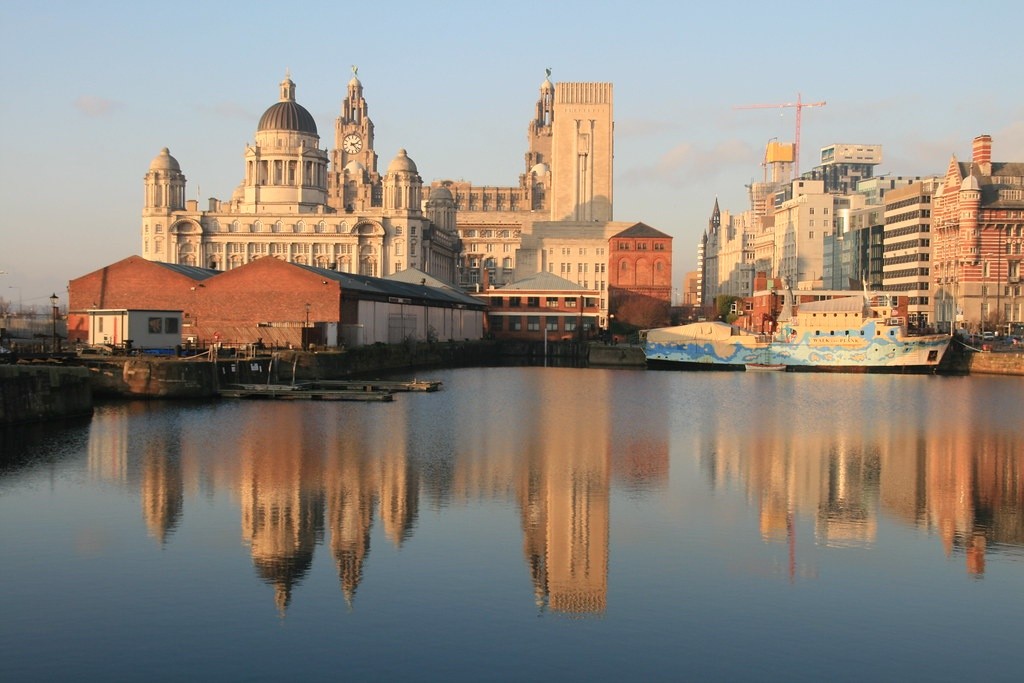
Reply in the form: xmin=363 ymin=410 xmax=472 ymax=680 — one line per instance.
xmin=639 ymin=312 xmax=952 ymax=368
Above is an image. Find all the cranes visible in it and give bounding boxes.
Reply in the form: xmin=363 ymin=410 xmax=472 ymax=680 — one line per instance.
xmin=733 ymin=92 xmax=827 ymax=179
